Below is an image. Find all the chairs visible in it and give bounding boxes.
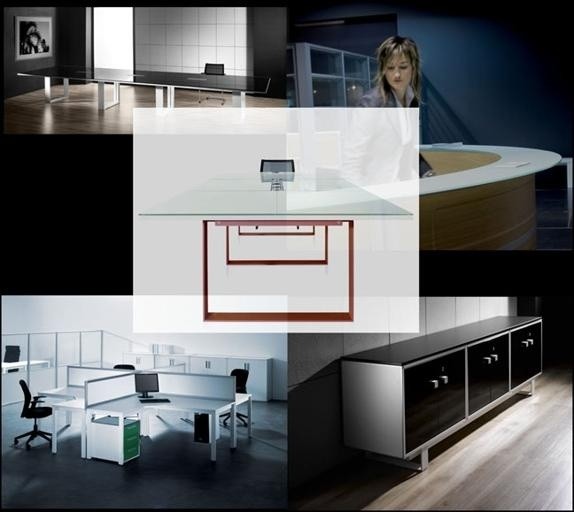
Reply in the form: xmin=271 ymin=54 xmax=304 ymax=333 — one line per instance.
xmin=4 ymin=346 xmax=20 ymax=373
xmin=14 ymin=379 xmax=52 ymax=450
xmin=113 ymin=365 xmax=135 ymax=370
xmin=198 ymin=63 xmax=226 ymax=105
xmin=219 ymin=369 xmax=249 ymax=427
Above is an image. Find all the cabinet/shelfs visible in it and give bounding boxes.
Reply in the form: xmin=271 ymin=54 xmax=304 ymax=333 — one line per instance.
xmin=127 ymin=352 xmax=273 ymax=402
xmin=287 ymin=42 xmax=379 ymax=107
xmin=340 ymin=320 xmax=542 ymax=472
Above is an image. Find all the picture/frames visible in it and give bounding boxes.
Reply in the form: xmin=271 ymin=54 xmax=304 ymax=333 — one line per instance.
xmin=15 ymin=15 xmax=53 ymax=62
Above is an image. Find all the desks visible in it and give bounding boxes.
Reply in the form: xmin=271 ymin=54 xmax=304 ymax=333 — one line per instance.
xmin=1 ymin=360 xmax=50 ymax=373
xmin=16 ymin=66 xmax=271 ymax=111
xmin=38 ymin=386 xmax=252 ymax=461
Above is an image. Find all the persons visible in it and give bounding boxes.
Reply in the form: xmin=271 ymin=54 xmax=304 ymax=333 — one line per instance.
xmin=348 ymin=32 xmax=437 ymax=183
